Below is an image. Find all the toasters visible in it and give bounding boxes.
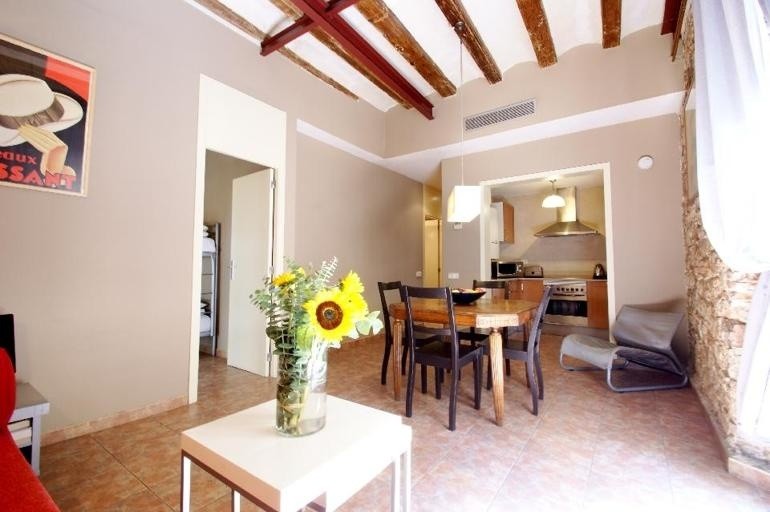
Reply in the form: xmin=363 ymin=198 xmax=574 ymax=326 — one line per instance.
xmin=525 ymin=266 xmax=543 ymax=278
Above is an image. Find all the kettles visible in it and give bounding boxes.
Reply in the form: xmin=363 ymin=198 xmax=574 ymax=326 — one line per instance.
xmin=593 ymin=264 xmax=605 ymax=277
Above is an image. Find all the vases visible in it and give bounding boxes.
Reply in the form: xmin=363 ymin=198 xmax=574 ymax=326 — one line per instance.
xmin=270 ymin=340 xmax=329 ymax=437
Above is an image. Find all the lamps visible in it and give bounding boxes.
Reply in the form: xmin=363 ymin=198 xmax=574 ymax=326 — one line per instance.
xmin=446 ymin=21 xmax=482 ymax=225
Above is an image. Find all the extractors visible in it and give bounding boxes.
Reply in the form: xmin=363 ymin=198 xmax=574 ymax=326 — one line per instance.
xmin=535 ymin=186 xmax=602 ymax=238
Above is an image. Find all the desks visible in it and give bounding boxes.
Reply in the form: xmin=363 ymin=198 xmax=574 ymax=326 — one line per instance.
xmin=179 ymin=391 xmax=414 ymax=512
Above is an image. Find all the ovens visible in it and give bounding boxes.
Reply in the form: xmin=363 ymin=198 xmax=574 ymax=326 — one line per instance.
xmin=543 ymin=279 xmax=587 ymax=325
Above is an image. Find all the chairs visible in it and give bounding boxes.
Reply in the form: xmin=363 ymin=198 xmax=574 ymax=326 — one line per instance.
xmin=378 ymin=276 xmax=553 ymax=432
xmin=559 ymin=302 xmax=690 ymax=394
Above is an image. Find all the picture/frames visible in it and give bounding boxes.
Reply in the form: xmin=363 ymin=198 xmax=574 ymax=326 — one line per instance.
xmin=0 ymin=32 xmax=97 ymax=198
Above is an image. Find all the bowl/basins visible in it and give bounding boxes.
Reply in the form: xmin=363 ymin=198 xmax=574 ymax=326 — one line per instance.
xmin=452 ymin=292 xmax=487 ymax=304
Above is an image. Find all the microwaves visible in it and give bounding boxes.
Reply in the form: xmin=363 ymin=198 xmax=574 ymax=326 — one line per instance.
xmin=491 ymin=258 xmax=524 ymax=278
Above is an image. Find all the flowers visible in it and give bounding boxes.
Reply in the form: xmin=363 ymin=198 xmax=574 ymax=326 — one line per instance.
xmin=247 ymin=255 xmax=384 ymax=432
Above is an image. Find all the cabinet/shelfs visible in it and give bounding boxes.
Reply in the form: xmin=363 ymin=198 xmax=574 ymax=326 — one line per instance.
xmin=491 ymin=280 xmax=543 ymax=332
xmin=490 ymin=201 xmax=515 ymax=245
xmin=586 ymin=282 xmax=608 ymax=330
